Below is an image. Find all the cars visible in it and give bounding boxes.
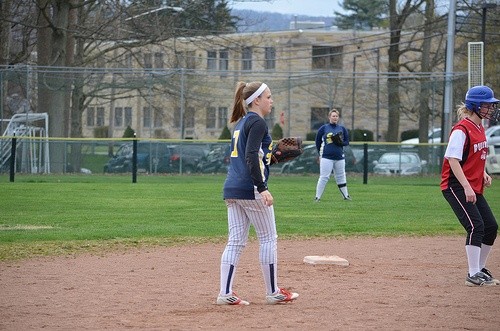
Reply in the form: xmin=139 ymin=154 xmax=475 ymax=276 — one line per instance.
xmin=485 ymin=125 xmax=500 ymax=147
xmin=373 ymin=152 xmax=430 ymax=177
xmin=400 ymin=127 xmax=442 ymax=148
xmin=104 ymin=141 xmax=173 ymax=174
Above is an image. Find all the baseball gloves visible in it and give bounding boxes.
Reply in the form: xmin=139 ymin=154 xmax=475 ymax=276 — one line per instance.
xmin=331 ymin=131 xmax=343 ymax=145
xmin=271 ymin=136 xmax=303 ymax=165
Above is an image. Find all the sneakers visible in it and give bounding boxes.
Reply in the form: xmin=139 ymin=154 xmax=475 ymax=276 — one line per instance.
xmin=465 ymin=268 xmax=500 ymax=287
xmin=266 ymin=288 xmax=299 ymax=305
xmin=217 ymin=291 xmax=249 ymax=306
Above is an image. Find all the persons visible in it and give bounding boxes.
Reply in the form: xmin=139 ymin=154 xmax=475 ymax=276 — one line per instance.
xmin=217 ymin=81 xmax=300 ymax=304
xmin=315 ymin=108 xmax=353 ymax=200
xmin=440 ymin=86 xmax=500 ymax=287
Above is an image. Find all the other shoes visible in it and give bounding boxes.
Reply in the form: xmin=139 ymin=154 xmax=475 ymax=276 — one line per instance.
xmin=344 ymin=195 xmax=352 ymax=201
xmin=315 ymin=197 xmax=320 ymax=202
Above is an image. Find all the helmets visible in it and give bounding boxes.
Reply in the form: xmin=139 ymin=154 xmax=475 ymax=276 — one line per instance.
xmin=465 ymin=86 xmax=500 ymax=112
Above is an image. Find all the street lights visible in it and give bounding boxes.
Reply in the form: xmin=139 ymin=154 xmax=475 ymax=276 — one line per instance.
xmin=374 ymin=48 xmax=380 ymax=143
xmin=287 ymin=29 xmax=304 ymax=136
xmin=124 ymin=5 xmax=184 ymax=21
xmin=351 ymin=53 xmax=362 ymax=141
xmin=480 ymin=1 xmax=497 ymax=45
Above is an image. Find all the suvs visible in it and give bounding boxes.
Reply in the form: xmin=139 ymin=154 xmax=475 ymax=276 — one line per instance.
xmin=152 ymin=143 xmax=210 ymax=175
xmin=196 ymin=142 xmax=230 ymax=173
xmin=346 ymin=147 xmax=384 ymax=172
xmin=281 ymin=142 xmax=357 ymax=176
